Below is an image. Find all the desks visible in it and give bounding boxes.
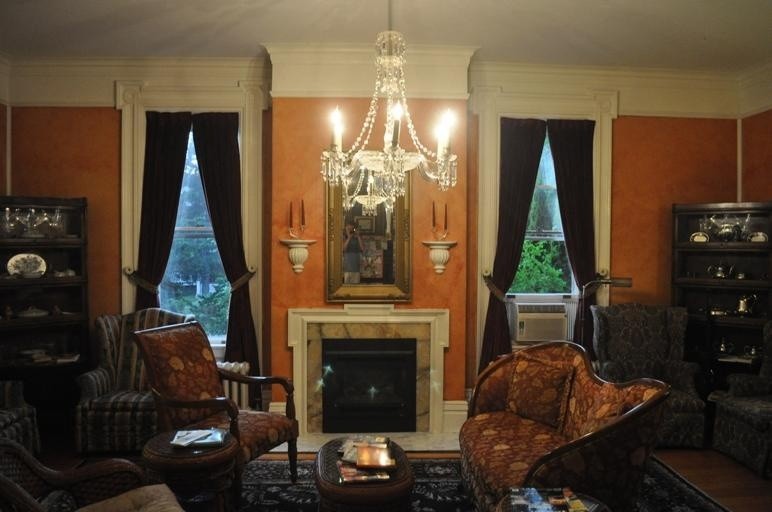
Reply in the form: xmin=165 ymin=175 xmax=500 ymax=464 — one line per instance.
xmin=143 ymin=426 xmax=240 ymax=511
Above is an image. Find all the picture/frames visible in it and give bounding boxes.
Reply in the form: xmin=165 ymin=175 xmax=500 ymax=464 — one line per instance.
xmin=324 ymin=149 xmax=415 ymax=305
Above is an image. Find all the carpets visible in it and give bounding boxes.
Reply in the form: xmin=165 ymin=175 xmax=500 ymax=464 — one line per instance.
xmin=240 ymin=455 xmax=731 ymax=512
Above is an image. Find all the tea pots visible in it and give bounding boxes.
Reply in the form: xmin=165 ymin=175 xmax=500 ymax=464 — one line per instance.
xmin=709 ymin=215 xmax=742 ymax=241
xmin=707 ymin=260 xmax=736 ymax=279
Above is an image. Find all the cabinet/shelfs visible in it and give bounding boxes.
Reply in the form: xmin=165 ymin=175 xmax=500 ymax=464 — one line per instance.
xmin=0 ymin=194 xmax=91 ymax=442
xmin=669 ymin=202 xmax=772 ymax=383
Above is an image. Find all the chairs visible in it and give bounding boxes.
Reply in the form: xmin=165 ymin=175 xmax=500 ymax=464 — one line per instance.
xmin=133 ymin=319 xmax=299 ymax=500
xmin=459 ymin=338 xmax=667 ymax=512
xmin=71 ymin=308 xmax=195 ymax=461
xmin=709 ymin=322 xmax=771 ymax=476
xmin=589 ymin=303 xmax=714 ymax=450
xmin=1 ymin=401 xmax=187 ymax=512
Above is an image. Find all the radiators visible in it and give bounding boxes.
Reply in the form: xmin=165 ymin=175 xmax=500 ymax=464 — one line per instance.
xmin=215 ymin=361 xmax=252 ymax=411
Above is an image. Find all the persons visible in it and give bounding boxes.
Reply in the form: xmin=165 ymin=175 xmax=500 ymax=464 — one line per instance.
xmin=344 ymin=222 xmax=366 ymax=284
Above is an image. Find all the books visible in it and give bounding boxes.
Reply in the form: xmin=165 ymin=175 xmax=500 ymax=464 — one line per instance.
xmin=338 ymin=434 xmax=396 ymax=482
xmin=172 ymin=430 xmax=224 ymax=447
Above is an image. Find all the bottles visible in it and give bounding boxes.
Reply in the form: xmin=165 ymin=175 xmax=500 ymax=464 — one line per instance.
xmin=719 ymin=336 xmax=728 ymax=353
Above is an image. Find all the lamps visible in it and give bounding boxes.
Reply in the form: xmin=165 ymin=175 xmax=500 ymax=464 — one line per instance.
xmin=581 ymin=278 xmax=632 ymax=346
xmin=430 ymin=200 xmax=448 ymax=241
xmin=320 ymin=1 xmax=459 ymax=217
xmin=286 ymin=199 xmax=306 ymax=240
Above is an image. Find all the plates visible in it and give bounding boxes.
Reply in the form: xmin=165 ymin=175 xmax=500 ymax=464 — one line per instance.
xmin=690 ymin=232 xmax=709 ymax=242
xmin=7 ymin=253 xmax=47 ymax=277
xmin=746 ymin=232 xmax=768 ymax=242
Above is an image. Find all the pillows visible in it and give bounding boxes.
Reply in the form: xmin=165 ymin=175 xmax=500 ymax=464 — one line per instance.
xmin=506 ymin=351 xmax=576 ymax=431
